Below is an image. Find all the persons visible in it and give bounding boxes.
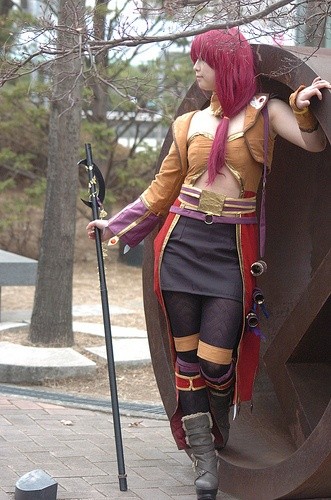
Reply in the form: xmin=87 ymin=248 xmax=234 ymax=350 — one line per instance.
xmin=85 ymin=27 xmax=331 ymax=500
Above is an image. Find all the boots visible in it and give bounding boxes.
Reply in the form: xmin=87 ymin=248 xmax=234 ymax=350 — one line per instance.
xmin=209 ymin=398 xmax=229 ymax=450
xmin=182 ymin=411 xmax=220 ymax=500
